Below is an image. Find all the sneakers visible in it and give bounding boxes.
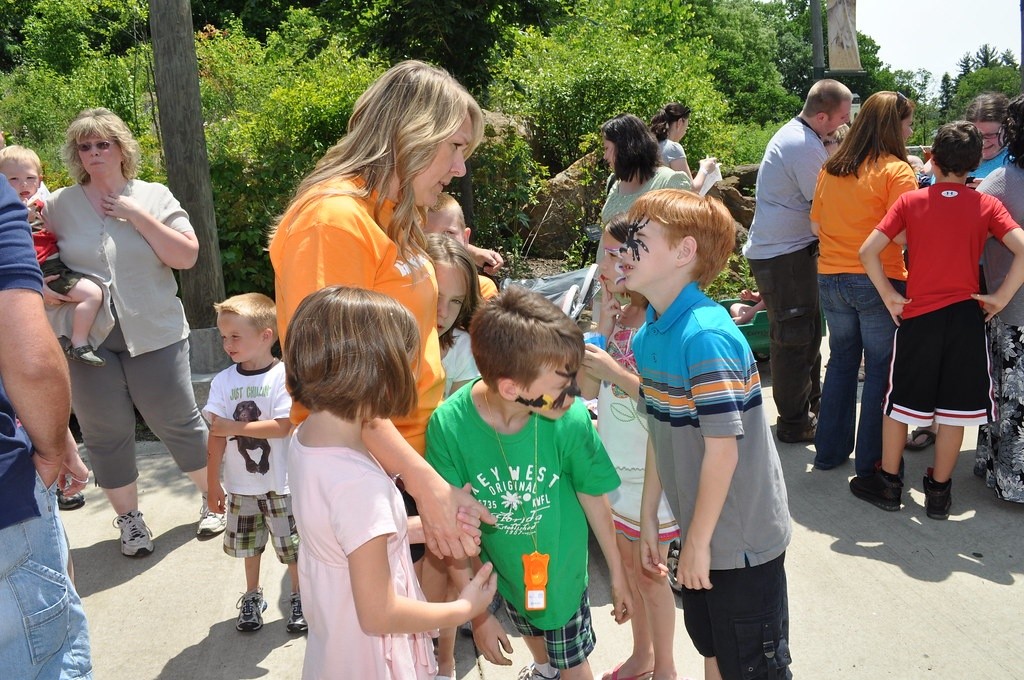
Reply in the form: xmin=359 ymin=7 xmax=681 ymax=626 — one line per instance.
xmin=923 ymin=467 xmax=951 ymax=520
xmin=850 ymin=460 xmax=904 ymax=510
xmin=198 ymin=485 xmax=228 ymax=536
xmin=286 ymin=584 xmax=308 ymax=632
xmin=112 ymin=510 xmax=154 ymax=557
xmin=236 ymin=586 xmax=268 ymax=633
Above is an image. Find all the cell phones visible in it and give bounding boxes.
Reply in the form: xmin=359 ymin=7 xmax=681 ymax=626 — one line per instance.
xmin=965 ymin=176 xmax=975 ymax=187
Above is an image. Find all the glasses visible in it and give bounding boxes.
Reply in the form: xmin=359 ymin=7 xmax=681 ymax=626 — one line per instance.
xmin=982 ymin=133 xmax=999 ymax=139
xmin=77 ymin=142 xmax=114 ymax=153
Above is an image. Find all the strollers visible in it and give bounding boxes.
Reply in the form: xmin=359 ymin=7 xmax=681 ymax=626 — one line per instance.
xmin=502 ymin=263 xmax=602 ymax=328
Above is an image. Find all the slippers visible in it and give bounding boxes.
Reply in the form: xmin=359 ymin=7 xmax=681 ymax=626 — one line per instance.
xmin=904 ymin=430 xmax=936 ymax=449
xmin=603 ymin=661 xmax=655 ymax=680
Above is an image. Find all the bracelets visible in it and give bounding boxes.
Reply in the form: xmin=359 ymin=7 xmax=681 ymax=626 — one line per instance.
xmin=700 ymin=167 xmax=709 ymax=175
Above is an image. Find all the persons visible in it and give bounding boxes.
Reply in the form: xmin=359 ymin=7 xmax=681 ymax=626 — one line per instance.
xmin=592 ymin=116 xmax=692 ymax=300
xmin=620 ymin=189 xmax=794 ymax=679
xmin=1 ymin=172 xmax=94 ymax=680
xmin=1 ymin=135 xmax=85 ymax=510
xmin=424 ymin=290 xmax=636 ymax=680
xmin=41 ymin=108 xmax=229 ymax=556
xmin=203 ymin=293 xmax=307 ymax=633
xmin=0 ymin=145 xmax=107 ymax=366
xmin=809 ymin=91 xmax=918 ymax=475
xmin=423 ymin=194 xmax=480 ymax=402
xmin=730 ymin=289 xmax=766 ymax=324
xmin=652 ymin=103 xmax=717 ymax=192
xmin=577 ymin=213 xmax=681 ymax=680
xmin=284 ymin=286 xmax=496 ymax=680
xmin=267 ymin=60 xmax=503 ymax=655
xmin=849 ymin=122 xmax=1024 ymax=519
xmin=823 ymin=95 xmax=1024 ymax=505
xmin=743 ymin=81 xmax=854 ymax=444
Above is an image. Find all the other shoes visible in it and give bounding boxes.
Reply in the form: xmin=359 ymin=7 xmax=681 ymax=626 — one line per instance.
xmin=777 ymin=413 xmax=818 ymax=443
xmin=57 ymin=491 xmax=86 ymax=509
xmin=458 ymin=592 xmax=502 ymax=636
xmin=516 ymin=663 xmax=560 ymax=680
xmin=64 ymin=341 xmax=106 ymax=366
